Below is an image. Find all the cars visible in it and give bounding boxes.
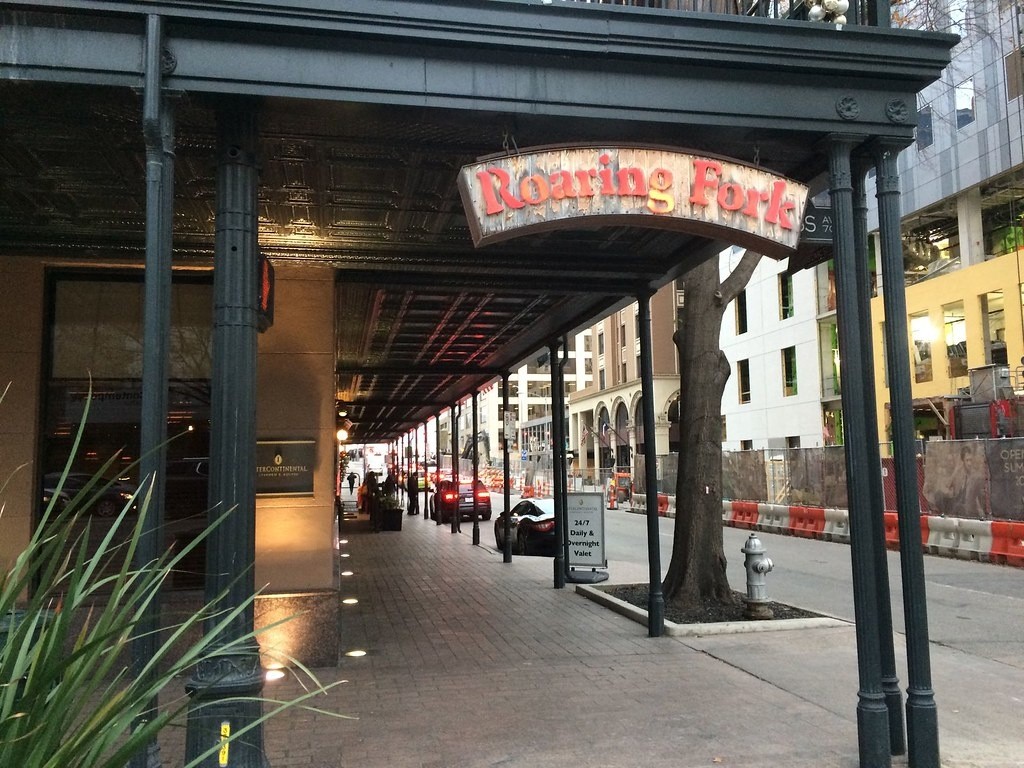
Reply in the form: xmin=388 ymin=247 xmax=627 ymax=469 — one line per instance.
xmin=44 ymin=472 xmax=139 ymax=519
xmin=396 ymin=464 xmax=453 ymax=493
xmin=494 ymin=497 xmax=554 ymax=557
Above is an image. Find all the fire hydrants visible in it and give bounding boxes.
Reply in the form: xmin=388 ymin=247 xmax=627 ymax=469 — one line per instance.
xmin=740 ymin=532 xmax=774 ymax=620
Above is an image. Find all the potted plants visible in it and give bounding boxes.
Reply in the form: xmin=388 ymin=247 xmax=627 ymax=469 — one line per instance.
xmin=381 ymin=495 xmax=404 ymax=531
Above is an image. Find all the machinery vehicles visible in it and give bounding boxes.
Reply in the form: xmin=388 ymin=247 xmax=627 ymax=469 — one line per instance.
xmin=607 ymin=472 xmax=635 ymax=503
xmin=949 ymin=399 xmax=1014 ymax=441
xmin=461 ymin=428 xmax=493 ymax=467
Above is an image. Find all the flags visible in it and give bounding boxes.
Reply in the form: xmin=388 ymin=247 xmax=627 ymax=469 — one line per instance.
xmin=599 ymin=417 xmax=610 ymax=447
xmin=581 ymin=423 xmax=589 ymax=447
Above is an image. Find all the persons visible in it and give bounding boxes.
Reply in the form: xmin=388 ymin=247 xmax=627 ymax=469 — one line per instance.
xmin=407 ymin=472 xmax=418 ymax=515
xmin=385 ymin=475 xmax=395 ymax=497
xmin=347 ymin=472 xmax=357 ymax=495
xmin=363 ymin=471 xmax=379 ymax=514
xmin=945 ymin=446 xmax=984 ymax=516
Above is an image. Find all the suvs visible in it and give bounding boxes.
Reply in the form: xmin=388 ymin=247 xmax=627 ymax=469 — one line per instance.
xmin=430 ymin=477 xmax=492 ymax=523
